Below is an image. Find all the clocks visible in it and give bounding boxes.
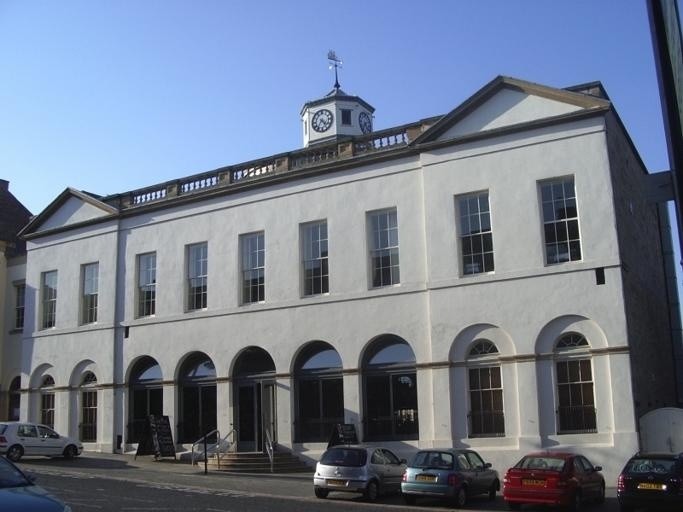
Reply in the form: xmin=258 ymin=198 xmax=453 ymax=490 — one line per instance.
xmin=358 ymin=111 xmax=372 ymax=135
xmin=312 ymin=109 xmax=333 ymax=132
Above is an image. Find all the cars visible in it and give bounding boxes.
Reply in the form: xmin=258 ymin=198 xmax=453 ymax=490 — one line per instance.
xmin=0 ymin=421 xmax=83 ymax=461
xmin=503 ymin=452 xmax=605 ymax=509
xmin=617 ymin=452 xmax=682 ymax=508
xmin=0 ymin=455 xmax=73 ymax=512
xmin=312 ymin=445 xmax=409 ymax=501
xmin=401 ymin=448 xmax=500 ymax=507
